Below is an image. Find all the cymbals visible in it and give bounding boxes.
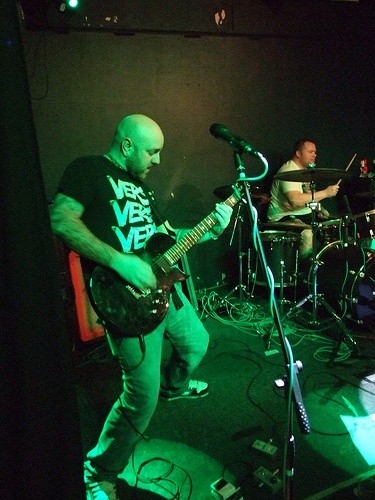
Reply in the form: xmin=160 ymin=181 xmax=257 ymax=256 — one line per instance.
xmin=263 ymin=219 xmax=312 ymax=231
xmin=212 ymin=183 xmax=270 ymax=206
xmin=274 ymin=167 xmax=353 ymax=182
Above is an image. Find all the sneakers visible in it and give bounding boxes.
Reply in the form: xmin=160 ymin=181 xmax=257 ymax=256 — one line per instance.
xmin=86 ymin=479 xmax=120 ymax=500
xmin=160 ymin=379 xmax=209 ymax=400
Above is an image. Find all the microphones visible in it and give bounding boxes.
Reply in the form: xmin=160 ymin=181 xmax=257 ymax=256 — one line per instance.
xmin=209 ymin=123 xmax=263 ymax=159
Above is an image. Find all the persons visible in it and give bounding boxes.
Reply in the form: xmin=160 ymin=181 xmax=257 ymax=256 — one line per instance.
xmin=267 ymin=140 xmax=339 ymax=266
xmin=51 ymin=113 xmax=233 ymax=500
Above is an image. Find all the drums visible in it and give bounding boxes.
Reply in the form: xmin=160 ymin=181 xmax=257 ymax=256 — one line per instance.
xmin=349 ymin=210 xmax=375 ymax=240
xmin=315 ymin=218 xmax=346 ymax=244
xmin=308 ymin=240 xmax=375 ymax=331
xmin=250 ymin=230 xmax=307 ymax=287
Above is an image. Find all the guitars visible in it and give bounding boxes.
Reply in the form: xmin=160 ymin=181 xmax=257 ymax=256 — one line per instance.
xmin=86 ymin=182 xmax=251 ymax=337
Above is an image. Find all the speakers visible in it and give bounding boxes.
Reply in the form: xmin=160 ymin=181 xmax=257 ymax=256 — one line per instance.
xmin=65 ymin=250 xmax=106 ymax=342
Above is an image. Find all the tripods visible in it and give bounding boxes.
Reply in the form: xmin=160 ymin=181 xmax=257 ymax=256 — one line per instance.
xmin=266 ymin=172 xmax=360 ymax=352
xmin=218 ymin=200 xmax=254 ymax=307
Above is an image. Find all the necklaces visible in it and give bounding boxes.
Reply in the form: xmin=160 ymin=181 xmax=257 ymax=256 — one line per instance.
xmin=109 ymin=155 xmax=125 ymax=171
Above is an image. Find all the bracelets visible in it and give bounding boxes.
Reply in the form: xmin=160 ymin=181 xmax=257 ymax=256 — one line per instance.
xmin=209 ymin=231 xmax=218 ymax=240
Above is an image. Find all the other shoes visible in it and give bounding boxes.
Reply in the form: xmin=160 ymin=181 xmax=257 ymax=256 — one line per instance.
xmin=303 ymin=279 xmax=318 ymax=286
